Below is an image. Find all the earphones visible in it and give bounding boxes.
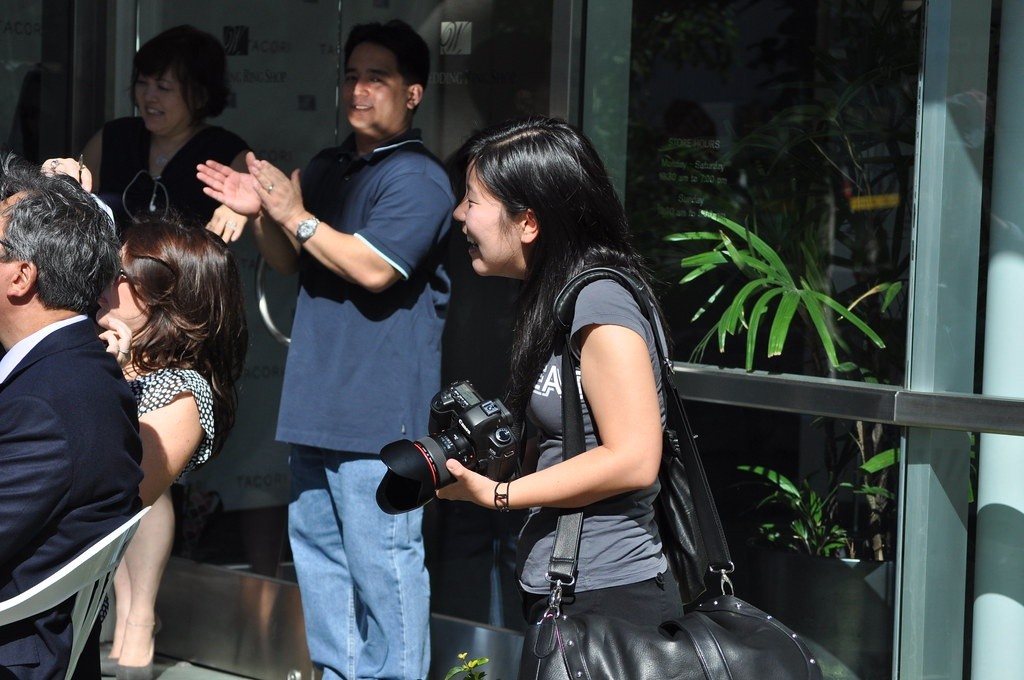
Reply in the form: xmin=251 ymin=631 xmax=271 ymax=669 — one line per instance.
xmin=149 ymin=194 xmax=157 ymax=212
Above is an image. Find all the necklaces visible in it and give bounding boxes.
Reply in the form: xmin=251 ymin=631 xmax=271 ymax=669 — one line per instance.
xmin=150 ymin=124 xmax=200 ymax=167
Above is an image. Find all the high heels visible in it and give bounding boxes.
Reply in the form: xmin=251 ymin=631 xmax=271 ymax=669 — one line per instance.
xmin=116 ymin=612 xmax=161 ymax=680
xmin=99 ymin=656 xmax=119 ymax=676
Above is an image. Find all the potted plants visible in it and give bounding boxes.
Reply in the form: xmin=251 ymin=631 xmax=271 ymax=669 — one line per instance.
xmin=661 ymin=208 xmax=977 ymax=680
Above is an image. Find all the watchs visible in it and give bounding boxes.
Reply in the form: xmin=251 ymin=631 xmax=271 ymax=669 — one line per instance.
xmin=295 ymin=217 xmax=320 ymax=243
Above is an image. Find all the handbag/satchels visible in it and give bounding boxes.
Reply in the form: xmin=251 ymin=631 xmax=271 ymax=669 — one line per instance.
xmin=520 ymin=595 xmax=824 ymax=680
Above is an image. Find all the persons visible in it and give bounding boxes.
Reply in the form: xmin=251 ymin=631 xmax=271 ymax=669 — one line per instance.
xmin=78 ymin=26 xmax=260 ymax=680
xmin=0 ymin=153 xmax=144 ymax=680
xmin=434 ymin=117 xmax=685 ymax=628
xmin=196 ymin=19 xmax=457 ymax=680
xmin=40 ymin=158 xmax=249 ymax=508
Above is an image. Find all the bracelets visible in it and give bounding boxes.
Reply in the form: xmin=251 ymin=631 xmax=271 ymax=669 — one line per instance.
xmin=494 ymin=482 xmax=511 ymax=512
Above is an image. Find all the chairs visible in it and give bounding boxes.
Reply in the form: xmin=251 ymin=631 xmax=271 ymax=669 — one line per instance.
xmin=0 ymin=506 xmax=153 ymax=680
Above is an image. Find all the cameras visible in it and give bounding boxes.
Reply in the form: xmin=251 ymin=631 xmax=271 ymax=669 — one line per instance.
xmin=375 ymin=375 xmax=517 ymax=515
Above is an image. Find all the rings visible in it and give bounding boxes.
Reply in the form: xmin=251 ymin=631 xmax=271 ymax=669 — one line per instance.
xmin=227 ymin=221 xmax=237 ymax=231
xmin=119 ymin=348 xmax=130 ymax=354
xmin=268 ymin=184 xmax=274 ymax=193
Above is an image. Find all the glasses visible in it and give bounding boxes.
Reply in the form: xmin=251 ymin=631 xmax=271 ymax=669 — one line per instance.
xmin=112 ymin=266 xmax=148 ymax=305
